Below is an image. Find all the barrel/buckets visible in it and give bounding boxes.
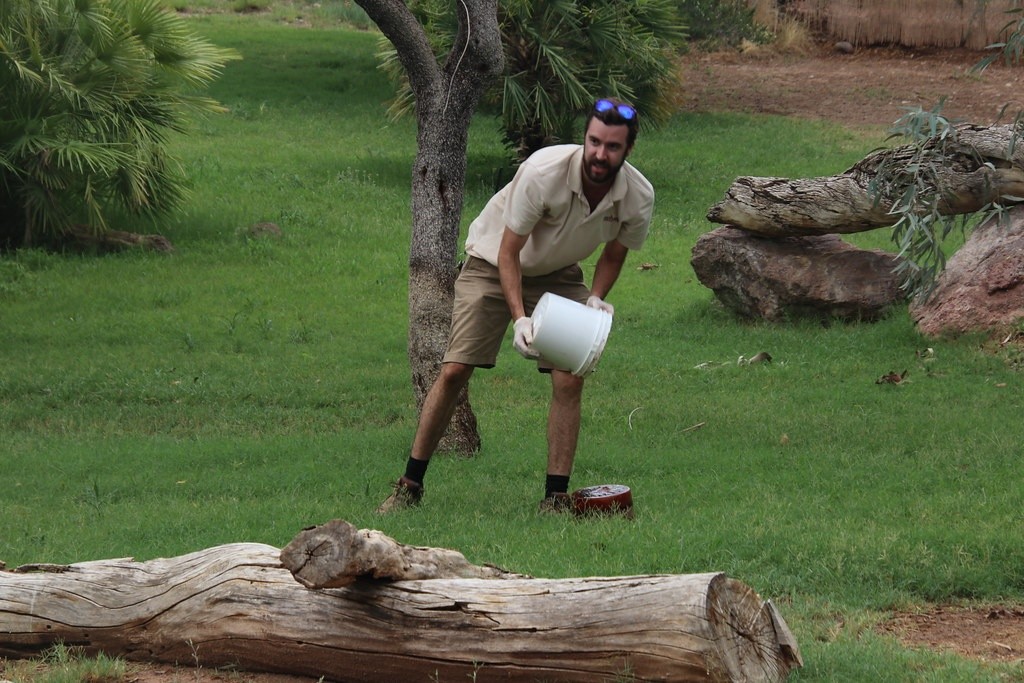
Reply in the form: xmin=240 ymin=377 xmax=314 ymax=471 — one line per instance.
xmin=524 ymin=292 xmax=613 ymax=379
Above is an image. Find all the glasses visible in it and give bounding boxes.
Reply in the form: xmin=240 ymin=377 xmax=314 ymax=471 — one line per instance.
xmin=595 ymin=100 xmax=638 ymax=120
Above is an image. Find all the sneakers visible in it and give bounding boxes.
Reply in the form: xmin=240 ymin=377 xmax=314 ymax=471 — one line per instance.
xmin=540 ymin=492 xmax=572 ymax=513
xmin=376 ymin=476 xmax=425 ymax=513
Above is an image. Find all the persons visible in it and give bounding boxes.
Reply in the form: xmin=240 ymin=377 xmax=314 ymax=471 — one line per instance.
xmin=374 ymin=97 xmax=656 ymax=519
